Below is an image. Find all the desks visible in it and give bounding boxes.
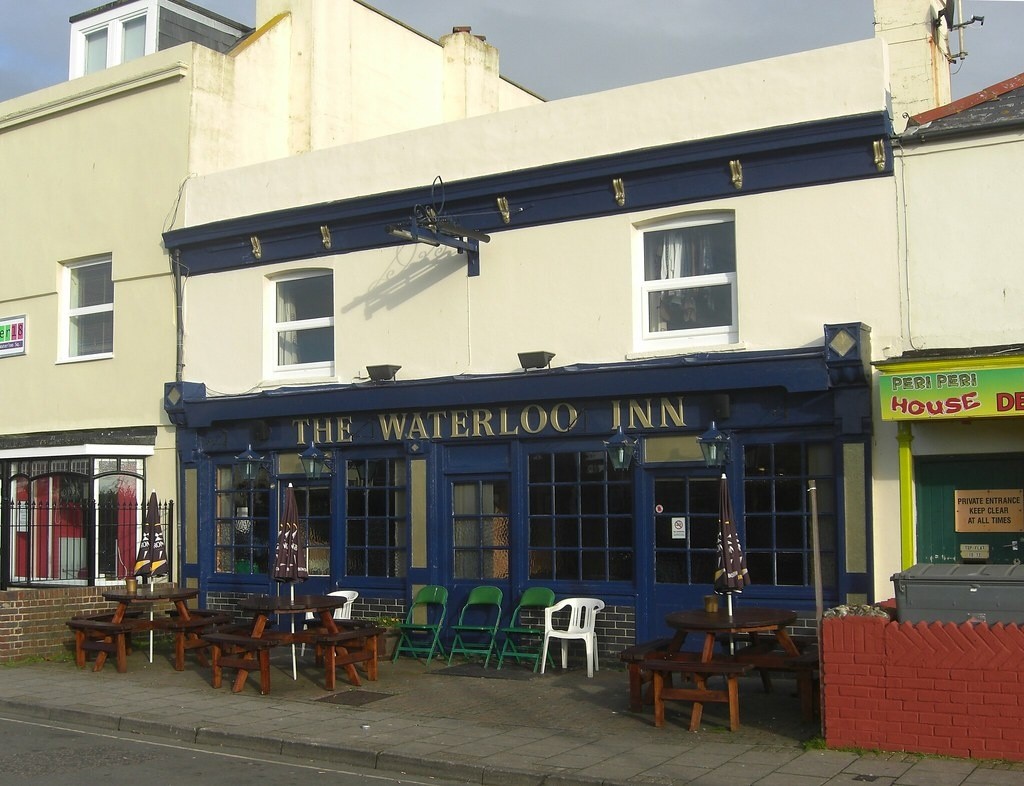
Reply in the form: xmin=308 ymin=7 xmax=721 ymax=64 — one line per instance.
xmin=642 ymin=606 xmax=803 ymax=732
xmin=93 ymin=588 xmax=210 ymax=672
xmin=232 ymin=595 xmax=361 ymax=692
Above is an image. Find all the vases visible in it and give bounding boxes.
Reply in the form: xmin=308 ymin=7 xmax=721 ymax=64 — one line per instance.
xmin=378 ymin=626 xmax=400 ymax=661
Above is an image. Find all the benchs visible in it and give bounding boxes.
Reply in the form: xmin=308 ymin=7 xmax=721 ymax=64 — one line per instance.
xmin=66 ymin=608 xmax=387 ymax=696
xmin=619 ymin=633 xmax=819 ymax=733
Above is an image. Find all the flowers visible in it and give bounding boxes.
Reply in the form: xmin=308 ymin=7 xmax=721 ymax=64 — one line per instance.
xmin=362 ymin=615 xmax=401 ymax=628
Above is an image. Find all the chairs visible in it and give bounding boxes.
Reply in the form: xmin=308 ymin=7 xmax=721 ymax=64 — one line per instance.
xmin=299 ymin=589 xmax=359 ymax=657
xmin=392 ymin=585 xmax=604 ymax=679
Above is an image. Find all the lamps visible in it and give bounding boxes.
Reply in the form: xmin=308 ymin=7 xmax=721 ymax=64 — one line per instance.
xmin=233 ymin=444 xmax=275 ymax=480
xmin=366 ymin=365 xmax=401 ymax=384
xmin=695 ymin=419 xmax=730 ymax=469
xmin=934 ymin=0 xmax=955 ymax=30
xmin=296 ymin=440 xmax=335 ymax=480
xmin=517 ymin=351 xmax=556 ymax=373
xmin=602 ymin=425 xmax=642 ymax=473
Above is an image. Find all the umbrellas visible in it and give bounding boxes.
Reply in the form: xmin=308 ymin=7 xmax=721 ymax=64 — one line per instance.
xmin=133 ymin=489 xmax=169 ymax=663
xmin=713 ymin=473 xmax=750 ymax=655
xmin=272 ymin=483 xmax=308 ymax=680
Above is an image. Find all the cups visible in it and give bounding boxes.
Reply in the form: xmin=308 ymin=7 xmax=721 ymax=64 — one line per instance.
xmin=126 ymin=577 xmax=137 ymax=592
xmin=705 ymin=595 xmax=718 ymax=612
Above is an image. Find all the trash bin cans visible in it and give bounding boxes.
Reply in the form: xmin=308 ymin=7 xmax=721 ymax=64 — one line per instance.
xmin=891 ymin=562 xmax=1024 ymax=628
